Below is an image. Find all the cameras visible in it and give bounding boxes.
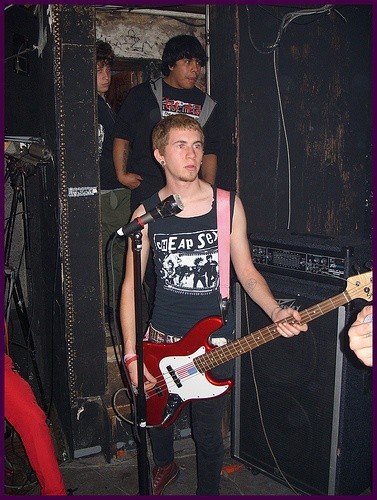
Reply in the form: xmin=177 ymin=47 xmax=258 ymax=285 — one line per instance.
xmin=3 ymin=134 xmax=52 ymax=167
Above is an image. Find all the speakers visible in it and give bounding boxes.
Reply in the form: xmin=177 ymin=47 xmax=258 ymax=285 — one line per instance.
xmin=230 ymin=228 xmax=373 ymax=496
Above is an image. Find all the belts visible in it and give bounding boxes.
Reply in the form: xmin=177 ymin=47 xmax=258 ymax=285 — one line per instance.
xmin=149 ymin=323 xmax=235 ymax=349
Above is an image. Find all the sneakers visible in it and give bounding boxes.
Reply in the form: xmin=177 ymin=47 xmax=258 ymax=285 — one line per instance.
xmin=134 ymin=461 xmax=180 ymax=496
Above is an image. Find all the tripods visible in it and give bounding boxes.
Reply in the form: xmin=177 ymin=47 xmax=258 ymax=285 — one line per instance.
xmin=4 ymin=170 xmax=51 ymax=439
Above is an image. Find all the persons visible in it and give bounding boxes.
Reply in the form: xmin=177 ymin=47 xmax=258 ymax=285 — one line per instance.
xmin=120 ymin=113 xmax=308 ymax=498
xmin=115 ymin=36 xmax=221 ymax=210
xmin=94 ymin=39 xmax=120 ymax=190
xmin=348 ymin=306 xmax=373 ymax=369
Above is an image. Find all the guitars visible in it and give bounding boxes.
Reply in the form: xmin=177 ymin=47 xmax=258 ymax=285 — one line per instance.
xmin=143 ymin=270 xmax=373 ymax=428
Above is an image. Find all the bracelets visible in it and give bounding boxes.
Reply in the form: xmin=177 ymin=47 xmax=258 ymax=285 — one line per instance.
xmin=125 ymin=356 xmax=136 ymax=367
xmin=124 ymin=353 xmax=136 ymax=363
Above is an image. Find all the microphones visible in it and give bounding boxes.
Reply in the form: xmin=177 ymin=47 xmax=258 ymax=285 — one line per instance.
xmin=115 ymin=192 xmax=184 ymax=236
